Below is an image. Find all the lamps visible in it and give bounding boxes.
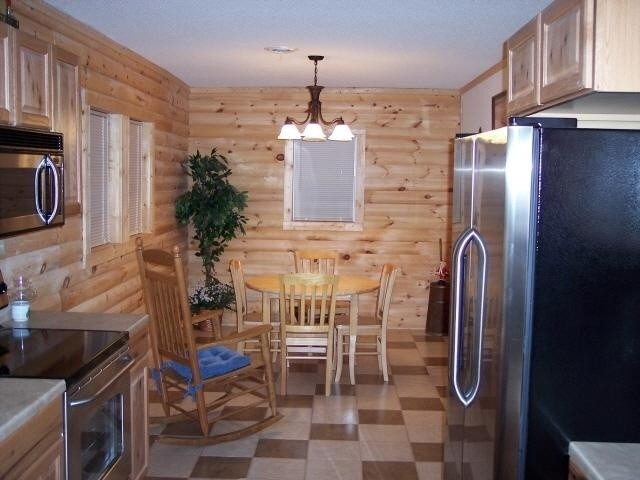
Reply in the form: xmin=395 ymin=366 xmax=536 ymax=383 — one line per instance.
xmin=276 ymin=55 xmax=355 ymax=143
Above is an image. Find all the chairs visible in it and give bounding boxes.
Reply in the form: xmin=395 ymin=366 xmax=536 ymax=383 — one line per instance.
xmin=229 ymin=258 xmax=290 ymax=377
xmin=277 ymin=273 xmax=341 ymax=397
xmin=334 ymin=263 xmax=398 ymax=384
xmin=288 ymin=249 xmax=341 ymax=357
xmin=134 ymin=235 xmax=285 ymax=447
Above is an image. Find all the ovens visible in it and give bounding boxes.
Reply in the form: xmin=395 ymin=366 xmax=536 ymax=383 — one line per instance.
xmin=64 ymin=343 xmax=133 ymax=480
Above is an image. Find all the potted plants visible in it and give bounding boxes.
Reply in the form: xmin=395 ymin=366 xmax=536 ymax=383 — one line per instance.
xmin=173 ymin=146 xmax=250 ymax=334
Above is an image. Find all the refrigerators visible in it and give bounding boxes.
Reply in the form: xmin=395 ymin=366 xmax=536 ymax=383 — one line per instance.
xmin=443 ymin=124 xmax=640 ymax=479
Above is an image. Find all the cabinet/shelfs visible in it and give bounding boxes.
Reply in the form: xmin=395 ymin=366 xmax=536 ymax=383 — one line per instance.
xmin=503 ymin=0 xmax=640 ymax=117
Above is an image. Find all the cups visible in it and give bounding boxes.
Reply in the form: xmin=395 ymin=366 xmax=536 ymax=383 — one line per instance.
xmin=9 ymin=274 xmax=37 ymax=323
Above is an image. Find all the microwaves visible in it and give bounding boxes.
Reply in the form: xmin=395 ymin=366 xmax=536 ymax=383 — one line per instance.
xmin=0 ymin=122 xmax=66 ymax=237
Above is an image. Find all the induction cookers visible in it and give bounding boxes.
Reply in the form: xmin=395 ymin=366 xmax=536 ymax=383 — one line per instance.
xmin=1 ymin=325 xmax=130 ymax=380
